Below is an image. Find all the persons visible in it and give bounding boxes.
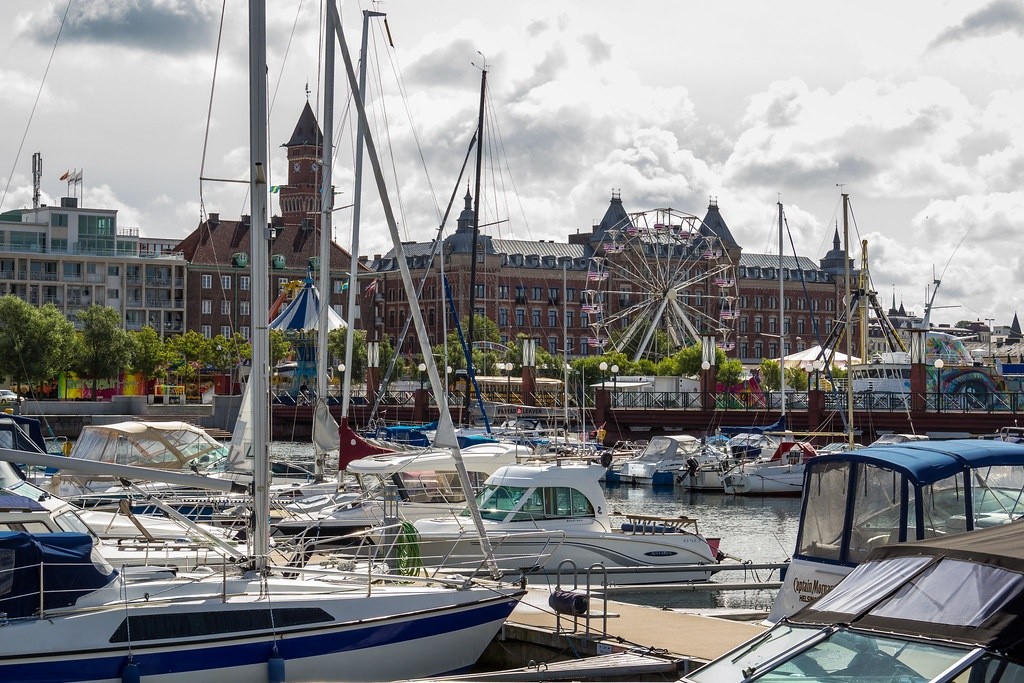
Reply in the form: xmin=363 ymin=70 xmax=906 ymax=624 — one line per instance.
xmin=9 ymin=376 xmax=91 ymax=401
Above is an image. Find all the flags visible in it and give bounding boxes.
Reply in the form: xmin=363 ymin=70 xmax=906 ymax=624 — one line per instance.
xmin=269 ymin=185 xmax=281 ymax=194
xmin=364 ymin=278 xmax=383 ymax=298
xmin=60 ymin=171 xmax=68 ymax=181
xmin=68 ymin=171 xmax=76 ymax=184
xmin=75 ymin=171 xmax=82 ymax=184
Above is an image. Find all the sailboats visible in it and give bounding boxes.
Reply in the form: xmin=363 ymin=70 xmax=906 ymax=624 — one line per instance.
xmin=2 ymin=2 xmax=1022 ymax=680
